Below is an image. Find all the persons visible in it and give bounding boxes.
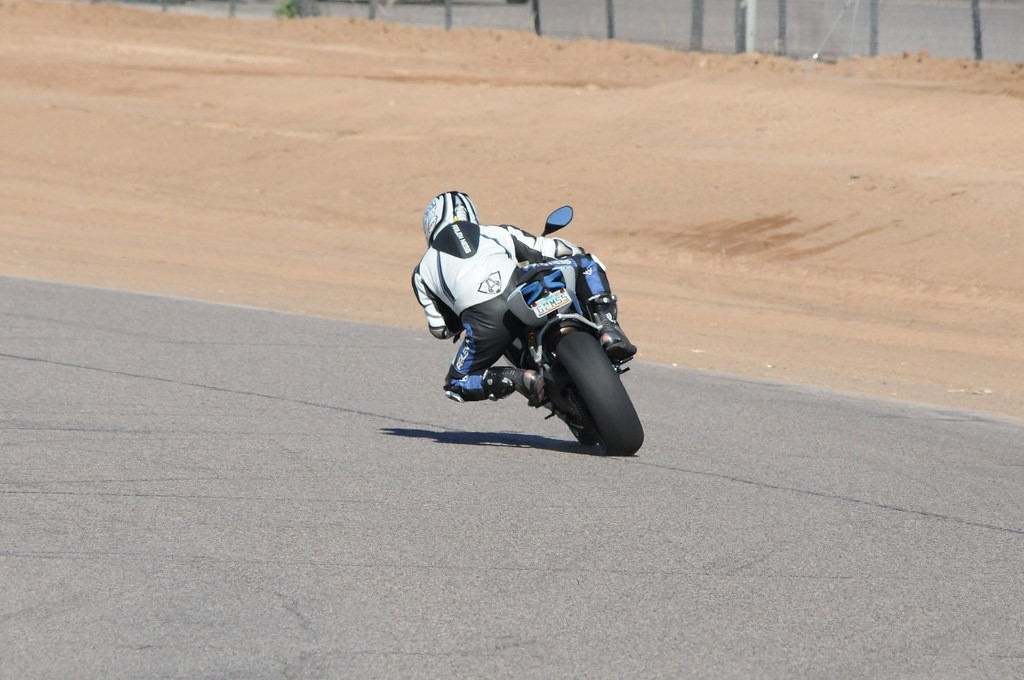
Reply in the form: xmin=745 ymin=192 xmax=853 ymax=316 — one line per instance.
xmin=412 ymin=191 xmax=637 ymax=407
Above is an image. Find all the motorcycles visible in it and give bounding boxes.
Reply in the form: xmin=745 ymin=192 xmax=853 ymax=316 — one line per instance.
xmin=453 ymin=205 xmax=645 ymax=458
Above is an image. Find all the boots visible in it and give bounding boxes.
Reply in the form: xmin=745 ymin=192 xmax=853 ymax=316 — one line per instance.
xmin=481 ymin=367 xmax=544 ymax=408
xmin=585 ymin=294 xmax=637 ymax=365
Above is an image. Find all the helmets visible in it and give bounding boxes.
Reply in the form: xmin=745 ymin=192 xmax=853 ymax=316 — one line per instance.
xmin=422 ymin=190 xmax=481 ymax=260
